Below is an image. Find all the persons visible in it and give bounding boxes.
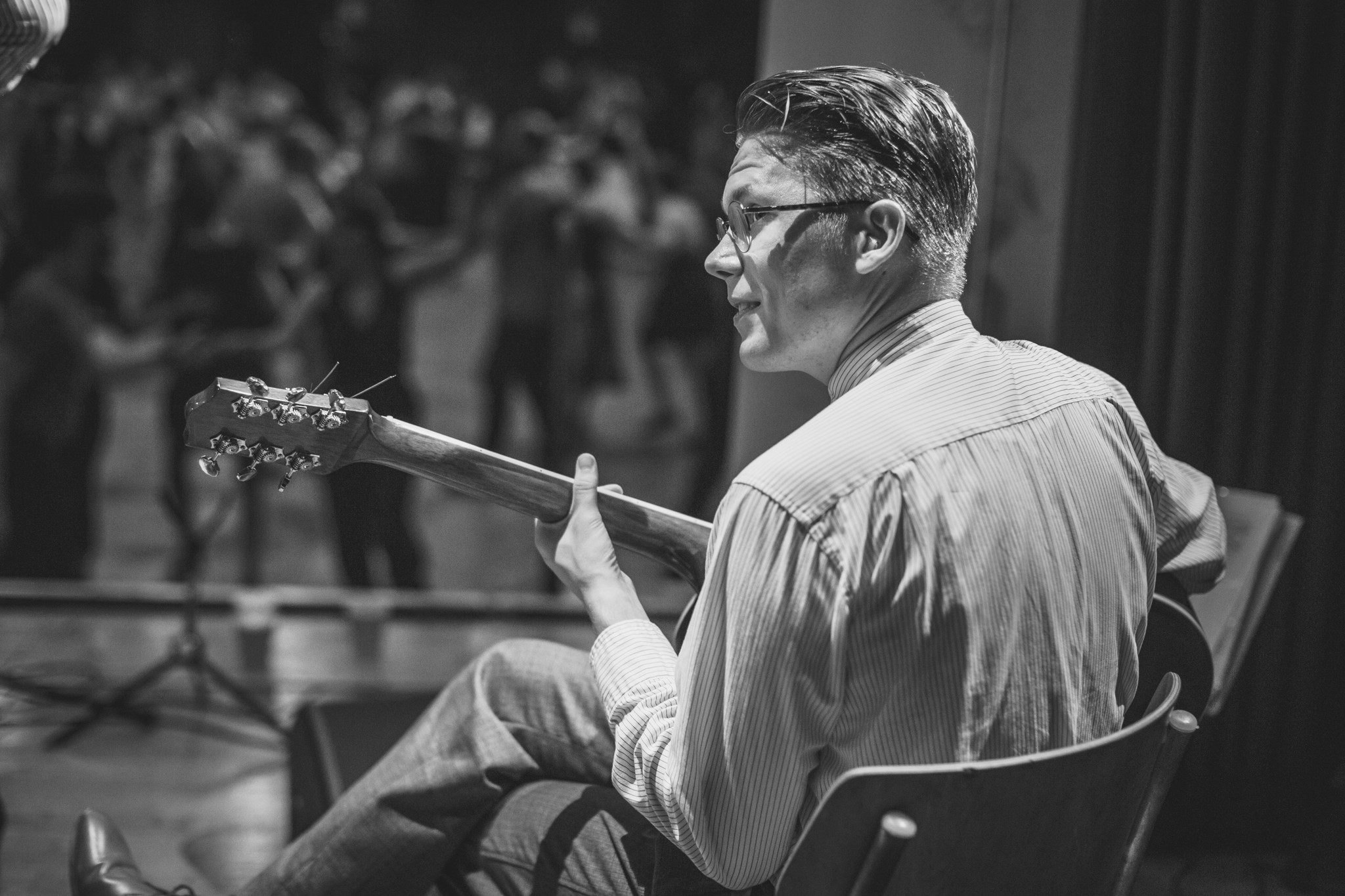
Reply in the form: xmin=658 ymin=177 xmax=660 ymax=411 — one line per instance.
xmin=1 ymin=36 xmax=733 ymax=593
xmin=70 ymin=59 xmax=1226 ymax=896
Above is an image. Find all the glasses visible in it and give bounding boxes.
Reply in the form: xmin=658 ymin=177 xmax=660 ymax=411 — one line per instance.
xmin=713 ymin=199 xmax=922 ymax=254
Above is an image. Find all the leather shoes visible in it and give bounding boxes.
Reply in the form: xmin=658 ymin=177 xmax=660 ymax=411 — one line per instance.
xmin=68 ymin=808 xmax=196 ymax=896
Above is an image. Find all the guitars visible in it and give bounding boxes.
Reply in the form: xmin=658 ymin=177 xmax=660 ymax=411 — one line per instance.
xmin=184 ymin=361 xmax=1216 ymax=731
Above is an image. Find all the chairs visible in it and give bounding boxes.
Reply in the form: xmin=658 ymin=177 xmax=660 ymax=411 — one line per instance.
xmin=773 ymin=672 xmax=1201 ymax=896
